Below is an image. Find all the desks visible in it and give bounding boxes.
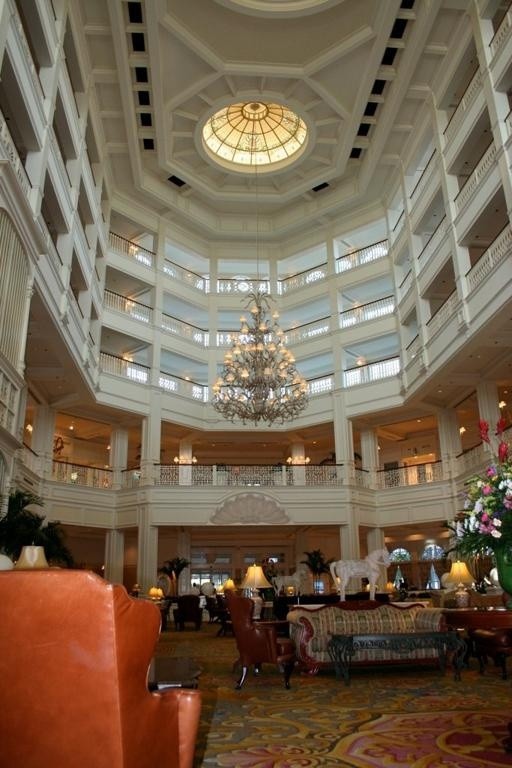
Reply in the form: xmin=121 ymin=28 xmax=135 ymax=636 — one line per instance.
xmin=440 ymin=607 xmax=512 ymax=679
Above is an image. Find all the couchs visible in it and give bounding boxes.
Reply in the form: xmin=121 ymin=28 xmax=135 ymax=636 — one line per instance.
xmin=286 ymin=598 xmax=468 ymax=681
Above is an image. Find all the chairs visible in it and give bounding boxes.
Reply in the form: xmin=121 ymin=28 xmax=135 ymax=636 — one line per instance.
xmin=173 ymin=595 xmax=204 ymax=631
xmin=223 ymin=595 xmax=297 ymax=690
xmin=205 ymin=594 xmax=233 ymax=638
xmin=470 ymin=628 xmax=512 ymax=677
xmin=1 ymin=569 xmax=202 ymax=768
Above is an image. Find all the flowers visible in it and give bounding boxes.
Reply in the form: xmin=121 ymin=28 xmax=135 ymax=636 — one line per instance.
xmin=438 ymin=413 xmax=512 ymax=556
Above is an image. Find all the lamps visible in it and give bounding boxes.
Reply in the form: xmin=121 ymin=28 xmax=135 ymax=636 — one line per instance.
xmin=12 ymin=545 xmax=50 ymax=570
xmin=210 ymin=110 xmax=311 ymax=428
xmin=240 ymin=563 xmax=273 ymax=620
xmin=442 ymin=561 xmax=475 ymax=606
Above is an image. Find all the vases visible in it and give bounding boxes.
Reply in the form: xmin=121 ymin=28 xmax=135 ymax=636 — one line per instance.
xmin=484 ymin=536 xmax=512 ymax=610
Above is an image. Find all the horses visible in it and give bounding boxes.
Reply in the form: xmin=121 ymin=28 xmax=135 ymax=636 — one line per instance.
xmin=329 ymin=546 xmax=391 ymax=602
